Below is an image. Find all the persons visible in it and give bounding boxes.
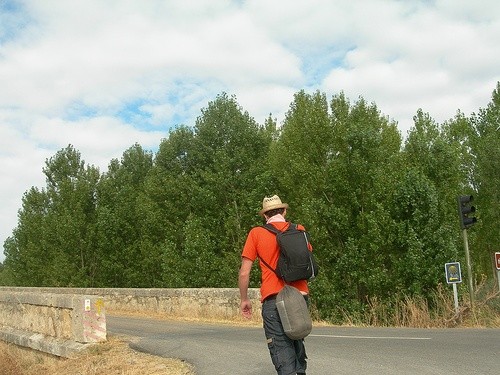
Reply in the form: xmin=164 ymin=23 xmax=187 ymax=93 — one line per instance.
xmin=238 ymin=195 xmax=313 ymax=375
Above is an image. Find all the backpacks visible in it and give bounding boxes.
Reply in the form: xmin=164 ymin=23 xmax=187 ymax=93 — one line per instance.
xmin=255 ymin=222 xmax=319 ymax=282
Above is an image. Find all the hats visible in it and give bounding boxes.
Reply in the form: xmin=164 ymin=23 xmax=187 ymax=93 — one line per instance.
xmin=259 ymin=195 xmax=288 ymax=216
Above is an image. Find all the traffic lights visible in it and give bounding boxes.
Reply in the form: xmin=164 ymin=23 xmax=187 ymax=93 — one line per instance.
xmin=457 ymin=192 xmax=477 ymax=234
